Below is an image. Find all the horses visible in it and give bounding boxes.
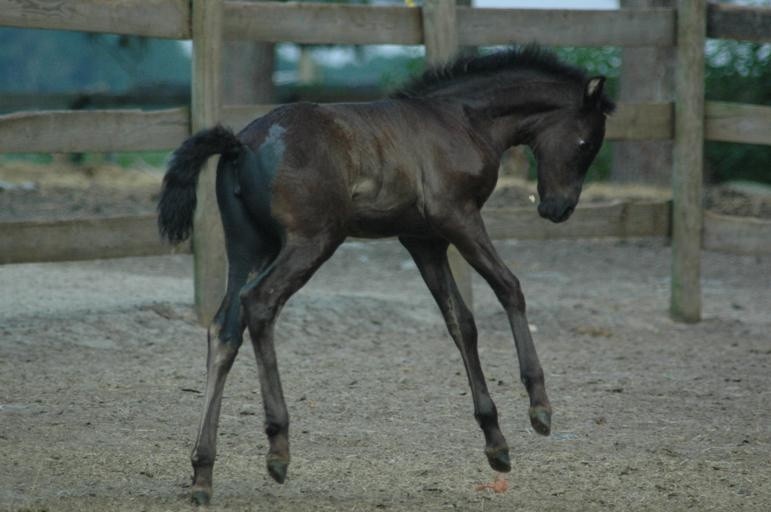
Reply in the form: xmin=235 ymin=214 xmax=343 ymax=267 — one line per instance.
xmin=157 ymin=43 xmax=616 ymax=506
